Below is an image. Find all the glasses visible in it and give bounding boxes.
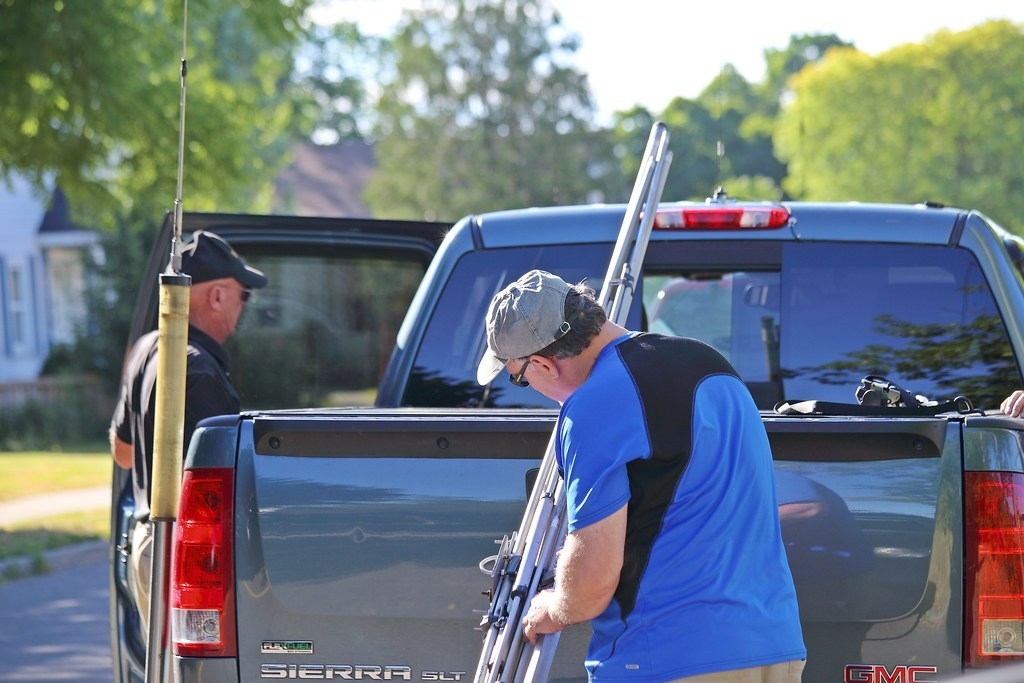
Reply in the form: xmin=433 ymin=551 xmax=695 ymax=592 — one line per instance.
xmin=509 ymin=356 xmax=530 ymax=387
xmin=216 ymin=283 xmax=251 ymax=302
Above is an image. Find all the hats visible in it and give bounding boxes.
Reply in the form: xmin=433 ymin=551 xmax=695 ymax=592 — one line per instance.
xmin=477 ymin=270 xmax=585 ymax=386
xmin=165 ymin=229 xmax=268 ymax=289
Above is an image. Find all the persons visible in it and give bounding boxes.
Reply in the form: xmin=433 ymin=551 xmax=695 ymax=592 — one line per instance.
xmin=1000 ymin=391 xmax=1024 ymax=419
xmin=773 ymin=466 xmax=872 ymax=622
xmin=475 ymin=270 xmax=808 ymax=683
xmin=109 ymin=230 xmax=269 ymax=633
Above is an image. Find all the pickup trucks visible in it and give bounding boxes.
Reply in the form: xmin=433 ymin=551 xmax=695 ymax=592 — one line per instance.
xmin=106 ymin=185 xmax=1023 ymax=683
